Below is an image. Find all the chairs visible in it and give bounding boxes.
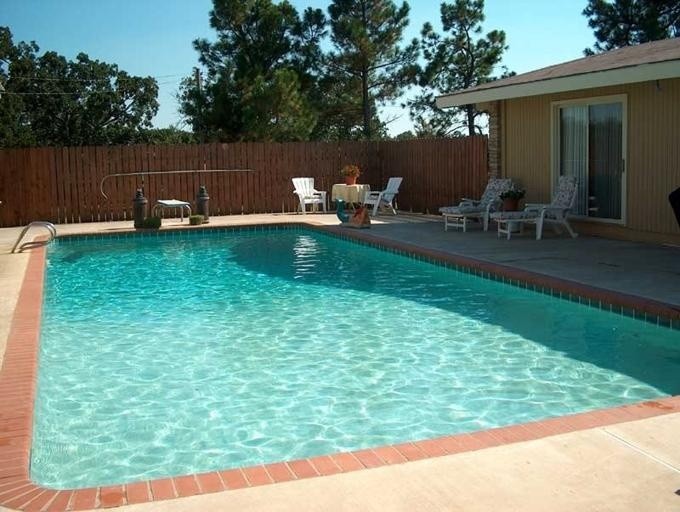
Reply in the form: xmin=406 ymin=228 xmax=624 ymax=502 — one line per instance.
xmin=440 ymin=176 xmax=516 ymax=232
xmin=490 ymin=175 xmax=579 ymax=240
xmin=291 ymin=176 xmax=328 ymax=216
xmin=362 ymin=176 xmax=404 ymax=217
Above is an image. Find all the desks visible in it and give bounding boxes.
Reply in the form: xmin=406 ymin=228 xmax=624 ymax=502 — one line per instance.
xmin=331 ymin=184 xmax=370 ymax=213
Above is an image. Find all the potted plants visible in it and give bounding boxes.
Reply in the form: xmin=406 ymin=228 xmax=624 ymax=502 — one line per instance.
xmin=500 ymin=188 xmax=526 ymax=211
xmin=340 ymin=164 xmax=361 ymax=184
